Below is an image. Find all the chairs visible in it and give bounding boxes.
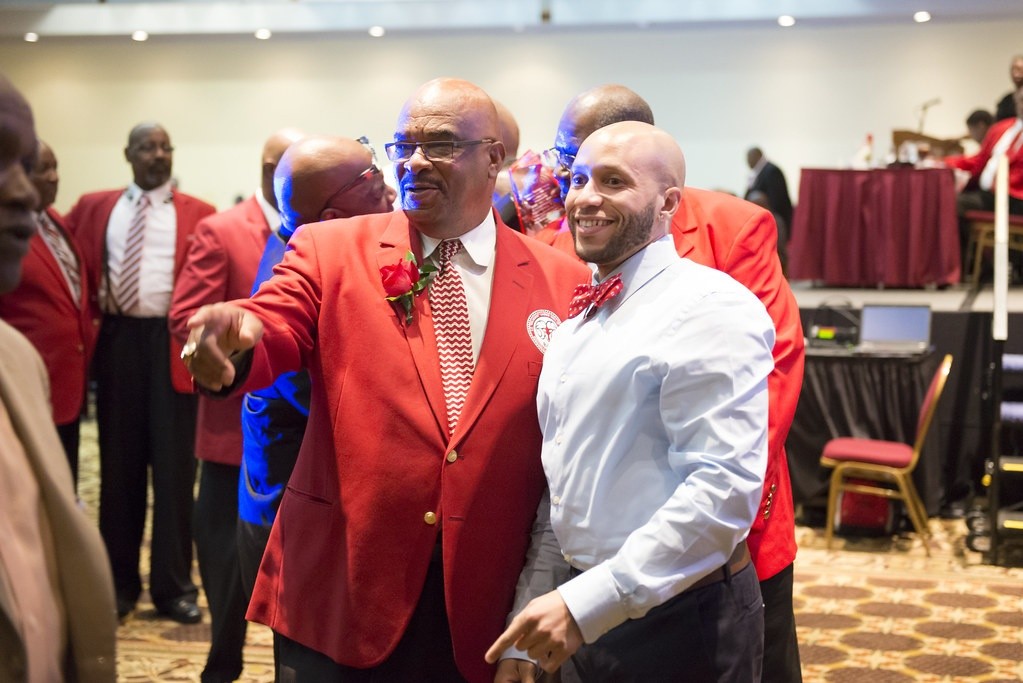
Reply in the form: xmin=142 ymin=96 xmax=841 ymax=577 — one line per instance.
xmin=819 ymin=352 xmax=953 ymax=559
xmin=962 ymin=209 xmax=1023 ymax=292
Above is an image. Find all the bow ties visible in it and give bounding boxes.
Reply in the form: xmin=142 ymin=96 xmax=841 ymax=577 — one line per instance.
xmin=568 ymin=272 xmax=624 ymax=317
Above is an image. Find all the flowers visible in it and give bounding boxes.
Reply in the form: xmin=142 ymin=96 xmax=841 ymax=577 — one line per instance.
xmin=379 ymin=250 xmax=439 ymax=325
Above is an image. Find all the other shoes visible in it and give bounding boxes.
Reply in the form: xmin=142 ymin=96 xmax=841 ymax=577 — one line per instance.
xmin=157 ymin=600 xmax=201 ymax=622
xmin=117 ymin=596 xmax=135 ymax=617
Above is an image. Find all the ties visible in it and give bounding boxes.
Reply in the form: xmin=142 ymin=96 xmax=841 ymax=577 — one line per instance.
xmin=116 ymin=195 xmax=173 ymax=313
xmin=428 ymin=242 xmax=474 ymax=444
xmin=39 ymin=217 xmax=82 ymax=301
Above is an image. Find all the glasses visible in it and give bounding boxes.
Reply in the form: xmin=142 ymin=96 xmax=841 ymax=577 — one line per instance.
xmin=325 ymin=136 xmax=381 ymax=210
xmin=385 ymin=138 xmax=494 ymax=162
xmin=543 ymin=147 xmax=575 ymax=166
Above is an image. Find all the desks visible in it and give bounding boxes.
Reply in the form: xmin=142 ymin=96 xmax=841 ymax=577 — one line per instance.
xmin=783 ymin=168 xmax=961 ymax=291
xmin=785 ymin=342 xmax=950 ymax=534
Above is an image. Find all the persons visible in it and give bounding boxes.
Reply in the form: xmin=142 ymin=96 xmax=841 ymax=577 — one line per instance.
xmin=932 ymin=55 xmax=1023 ymax=284
xmin=0 ymin=78 xmax=120 ymax=682
xmin=162 ymin=85 xmax=805 ymax=683
xmin=61 ymin=122 xmax=218 ymax=623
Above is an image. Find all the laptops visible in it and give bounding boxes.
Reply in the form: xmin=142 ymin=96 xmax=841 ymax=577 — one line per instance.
xmin=852 ymin=303 xmax=931 ymax=359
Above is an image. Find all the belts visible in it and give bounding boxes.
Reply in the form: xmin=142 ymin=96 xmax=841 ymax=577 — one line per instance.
xmin=686 ymin=539 xmax=751 ymax=591
